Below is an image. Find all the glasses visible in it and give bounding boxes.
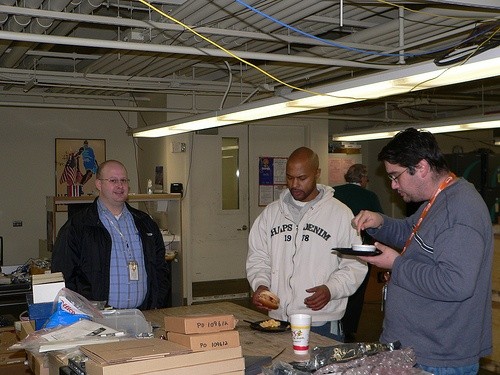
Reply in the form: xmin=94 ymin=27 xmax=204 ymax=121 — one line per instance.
xmin=387 ymin=167 xmax=408 ymax=183
xmin=99 ymin=177 xmax=130 ymax=185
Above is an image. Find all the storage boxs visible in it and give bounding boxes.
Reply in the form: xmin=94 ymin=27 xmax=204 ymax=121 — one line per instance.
xmin=29 ymin=269 xmax=245 ymax=375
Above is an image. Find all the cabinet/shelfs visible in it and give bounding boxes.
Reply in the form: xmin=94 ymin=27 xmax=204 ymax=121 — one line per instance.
xmin=46 ymin=193 xmax=183 ymax=259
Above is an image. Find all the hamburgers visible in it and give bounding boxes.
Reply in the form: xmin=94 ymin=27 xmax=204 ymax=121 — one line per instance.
xmin=258 ymin=290 xmax=280 ymax=309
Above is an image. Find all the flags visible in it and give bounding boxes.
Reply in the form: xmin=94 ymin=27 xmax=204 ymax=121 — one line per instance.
xmin=60 ymin=159 xmax=77 ymax=184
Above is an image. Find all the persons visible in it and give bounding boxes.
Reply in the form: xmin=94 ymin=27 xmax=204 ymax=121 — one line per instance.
xmin=246 ymin=147 xmax=368 ymax=341
xmin=351 ymin=128 xmax=493 ymax=375
xmin=51 ymin=160 xmax=169 ymax=311
xmin=333 ymin=165 xmax=382 ymax=343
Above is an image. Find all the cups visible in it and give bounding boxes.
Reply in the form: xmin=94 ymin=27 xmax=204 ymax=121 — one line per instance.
xmin=291 ymin=314 xmax=311 ymax=355
xmin=352 ymin=244 xmax=376 ymax=251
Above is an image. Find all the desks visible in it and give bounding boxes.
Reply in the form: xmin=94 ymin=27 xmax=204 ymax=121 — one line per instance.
xmin=20 ymin=301 xmax=345 ymax=375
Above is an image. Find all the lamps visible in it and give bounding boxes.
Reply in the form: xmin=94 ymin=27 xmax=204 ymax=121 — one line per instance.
xmin=332 ymin=110 xmax=500 ymax=141
xmin=128 ymin=45 xmax=500 ymax=136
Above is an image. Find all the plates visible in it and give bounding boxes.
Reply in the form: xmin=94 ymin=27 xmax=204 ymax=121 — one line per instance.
xmin=331 ymin=248 xmax=382 ymax=256
xmin=249 ymin=319 xmax=290 ymax=333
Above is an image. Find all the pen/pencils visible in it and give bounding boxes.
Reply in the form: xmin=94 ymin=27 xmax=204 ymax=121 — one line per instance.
xmin=97 ymin=331 xmax=125 ymax=337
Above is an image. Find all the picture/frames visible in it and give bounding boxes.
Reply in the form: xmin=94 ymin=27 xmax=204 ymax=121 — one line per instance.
xmin=55 ymin=138 xmax=106 ymax=212
